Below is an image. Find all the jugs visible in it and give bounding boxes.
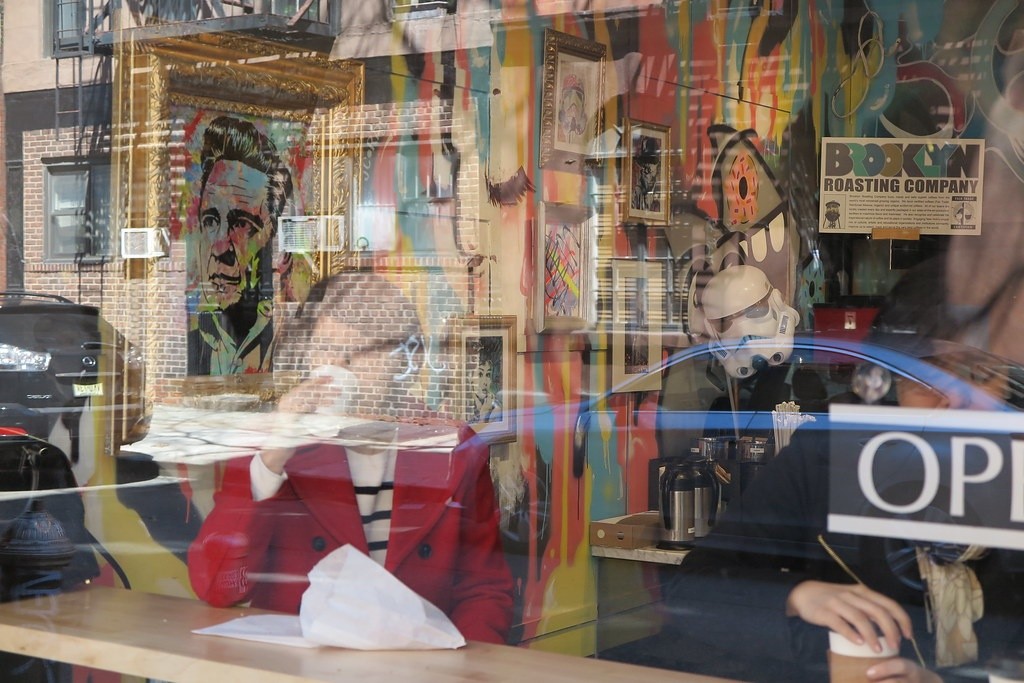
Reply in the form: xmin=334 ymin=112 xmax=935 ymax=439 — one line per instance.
xmin=656 ymin=455 xmax=719 ymax=551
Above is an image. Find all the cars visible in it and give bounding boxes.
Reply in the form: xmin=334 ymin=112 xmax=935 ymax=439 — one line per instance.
xmin=447 ymin=332 xmax=1024 ymax=605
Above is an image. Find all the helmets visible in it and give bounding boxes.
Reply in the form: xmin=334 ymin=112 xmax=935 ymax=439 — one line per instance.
xmin=701 ymin=265 xmax=800 ymax=378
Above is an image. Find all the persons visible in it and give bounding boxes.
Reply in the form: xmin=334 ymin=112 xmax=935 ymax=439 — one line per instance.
xmin=187 ymin=266 xmax=513 ymax=643
xmin=700 ymin=265 xmax=800 ymax=379
xmin=663 ymin=257 xmax=1023 ymax=683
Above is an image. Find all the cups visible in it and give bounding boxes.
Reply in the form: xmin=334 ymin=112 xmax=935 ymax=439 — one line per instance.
xmin=300 ymin=364 xmax=358 ymax=439
xmin=690 ymin=437 xmax=766 ymax=464
xmin=827 ymin=629 xmax=901 ymax=683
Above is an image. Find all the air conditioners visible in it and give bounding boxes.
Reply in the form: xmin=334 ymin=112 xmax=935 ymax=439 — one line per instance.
xmin=121 ymin=227 xmax=168 ymax=258
xmin=278 ymin=215 xmax=344 ymax=253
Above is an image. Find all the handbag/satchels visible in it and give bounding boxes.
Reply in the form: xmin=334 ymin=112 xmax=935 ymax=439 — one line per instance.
xmin=0 ymin=435 xmax=101 ymax=603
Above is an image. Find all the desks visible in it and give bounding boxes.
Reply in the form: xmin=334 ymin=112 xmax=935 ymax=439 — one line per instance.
xmin=0 ymin=587 xmax=738 ymax=683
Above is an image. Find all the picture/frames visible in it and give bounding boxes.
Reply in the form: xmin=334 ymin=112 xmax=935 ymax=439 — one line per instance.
xmin=605 ymin=258 xmax=663 ymax=396
xmin=623 ymin=117 xmax=671 ymax=227
xmin=539 ymin=27 xmax=607 ymax=177
xmin=533 ymin=201 xmax=589 ymax=335
xmin=445 ymin=314 xmax=518 ymax=445
xmin=106 ymin=9 xmax=363 ymax=458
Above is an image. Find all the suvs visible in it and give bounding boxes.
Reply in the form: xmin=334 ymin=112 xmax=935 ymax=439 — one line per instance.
xmin=0 ymin=290 xmax=152 ymax=453
